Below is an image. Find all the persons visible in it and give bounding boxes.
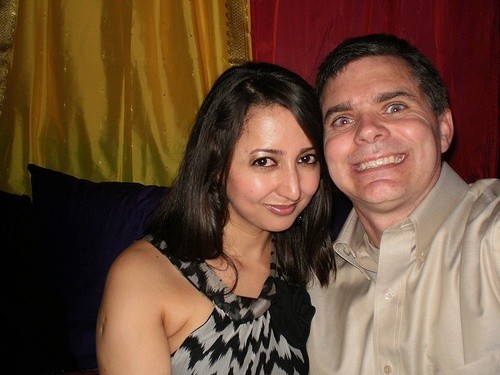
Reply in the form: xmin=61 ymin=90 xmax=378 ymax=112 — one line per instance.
xmin=305 ymin=31 xmax=500 ymax=375
xmin=94 ymin=60 xmax=338 ymax=375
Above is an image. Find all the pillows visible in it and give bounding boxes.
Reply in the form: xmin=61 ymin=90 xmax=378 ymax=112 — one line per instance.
xmin=27 ymin=163 xmax=173 ymax=372
